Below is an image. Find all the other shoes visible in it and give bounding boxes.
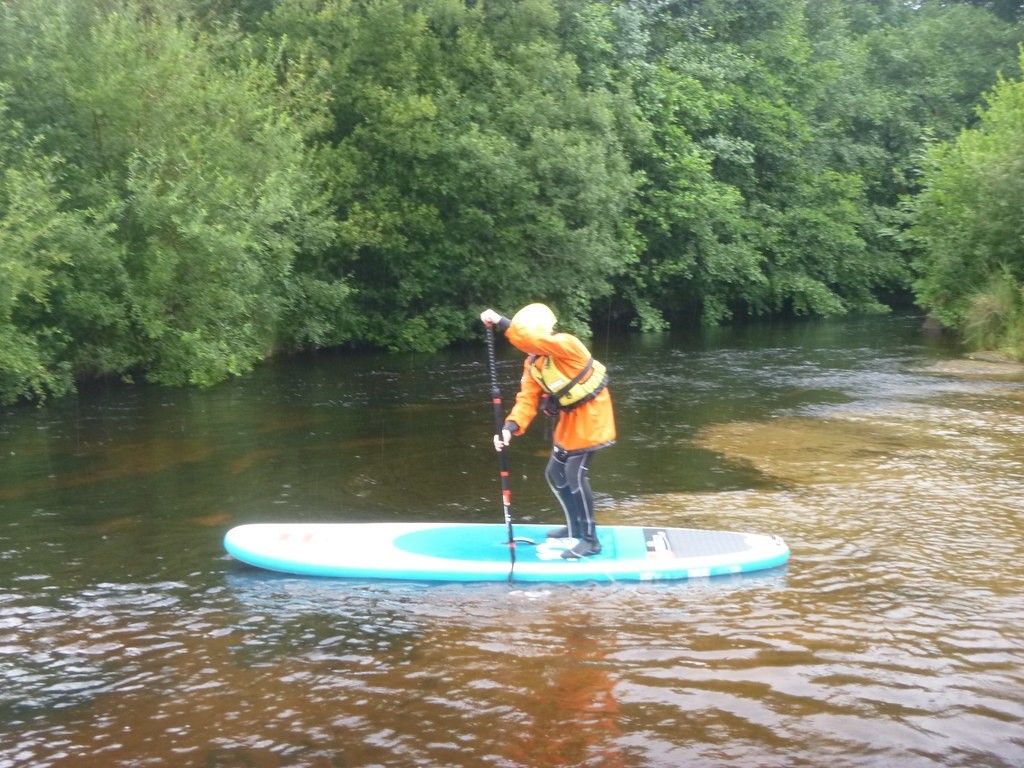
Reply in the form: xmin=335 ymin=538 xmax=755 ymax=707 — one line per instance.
xmin=561 ymin=539 xmax=602 ymax=559
xmin=547 ymin=526 xmax=568 ymax=538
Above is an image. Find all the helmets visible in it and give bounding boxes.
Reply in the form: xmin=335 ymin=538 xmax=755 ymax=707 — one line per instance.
xmin=510 ymin=303 xmax=557 ymax=334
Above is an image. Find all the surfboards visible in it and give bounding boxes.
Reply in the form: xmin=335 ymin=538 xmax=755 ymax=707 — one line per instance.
xmin=223 ymin=521 xmax=791 ymax=583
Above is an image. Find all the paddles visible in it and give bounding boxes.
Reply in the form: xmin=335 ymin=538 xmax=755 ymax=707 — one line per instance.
xmin=482 ymin=320 xmax=516 ymax=588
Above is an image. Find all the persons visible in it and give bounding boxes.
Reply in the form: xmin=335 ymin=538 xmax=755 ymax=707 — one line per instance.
xmin=480 ymin=303 xmax=616 ymax=559
